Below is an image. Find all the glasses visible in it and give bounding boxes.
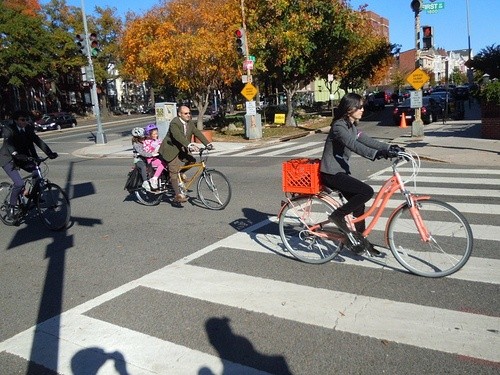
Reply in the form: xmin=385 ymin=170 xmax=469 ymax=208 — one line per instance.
xmin=354 ymin=105 xmax=363 ymax=109
xmin=182 ymin=113 xmax=190 ymax=114
xmin=18 ymin=118 xmax=29 ymax=122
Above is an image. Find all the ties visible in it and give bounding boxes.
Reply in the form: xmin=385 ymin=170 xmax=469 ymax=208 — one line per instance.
xmin=21 ymin=129 xmax=24 ymax=134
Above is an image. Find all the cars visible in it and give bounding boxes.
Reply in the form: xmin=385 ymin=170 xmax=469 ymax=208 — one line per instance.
xmin=368 ymin=81 xmax=474 ymax=125
xmin=32 ymin=112 xmax=77 ymax=132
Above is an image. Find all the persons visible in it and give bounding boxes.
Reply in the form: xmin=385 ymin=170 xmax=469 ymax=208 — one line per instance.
xmin=0 ymin=111 xmax=58 ymax=214
xmin=320 ymin=94 xmax=401 ymax=257
xmin=159 ymin=105 xmax=212 ymax=203
xmin=131 ymin=124 xmax=164 ymax=192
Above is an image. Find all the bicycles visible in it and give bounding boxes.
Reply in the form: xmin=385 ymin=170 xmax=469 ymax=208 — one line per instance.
xmin=277 ymin=147 xmax=474 ymax=278
xmin=125 ymin=144 xmax=232 ymax=212
xmin=0 ymin=155 xmax=72 ymax=232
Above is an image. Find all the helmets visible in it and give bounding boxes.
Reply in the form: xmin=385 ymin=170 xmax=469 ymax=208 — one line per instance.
xmin=145 ymin=125 xmax=158 ymax=136
xmin=132 ymin=127 xmax=144 ymax=137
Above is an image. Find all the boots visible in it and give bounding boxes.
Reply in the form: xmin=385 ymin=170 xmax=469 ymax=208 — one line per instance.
xmin=328 ymin=208 xmax=349 ymax=233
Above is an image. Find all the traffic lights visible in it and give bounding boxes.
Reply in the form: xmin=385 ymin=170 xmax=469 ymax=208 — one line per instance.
xmin=76 ymin=33 xmax=88 ymax=59
xmin=422 ymin=25 xmax=432 ymax=50
xmin=411 ymin=0 xmax=423 ymax=13
xmin=88 ymin=33 xmax=99 ymax=58
xmin=235 ymin=29 xmax=248 ymax=58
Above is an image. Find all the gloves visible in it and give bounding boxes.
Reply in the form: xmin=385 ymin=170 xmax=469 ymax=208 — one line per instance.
xmin=374 ymin=149 xmax=394 ymax=160
xmin=48 ymin=152 xmax=57 ymax=159
xmin=391 ymin=145 xmax=404 ymax=151
xmin=13 ymin=153 xmax=27 ymax=161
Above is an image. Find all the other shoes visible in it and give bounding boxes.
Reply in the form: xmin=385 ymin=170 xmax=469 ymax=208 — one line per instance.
xmin=149 ymin=178 xmax=158 ymax=189
xmin=174 ymin=194 xmax=189 ymax=201
xmin=142 ymin=181 xmax=151 ymax=191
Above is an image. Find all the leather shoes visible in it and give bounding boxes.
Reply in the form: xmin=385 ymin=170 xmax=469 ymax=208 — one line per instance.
xmin=5 ymin=205 xmax=16 ymax=221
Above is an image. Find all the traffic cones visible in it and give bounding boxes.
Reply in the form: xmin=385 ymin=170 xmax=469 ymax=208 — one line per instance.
xmin=398 ymin=112 xmax=409 ymax=128
